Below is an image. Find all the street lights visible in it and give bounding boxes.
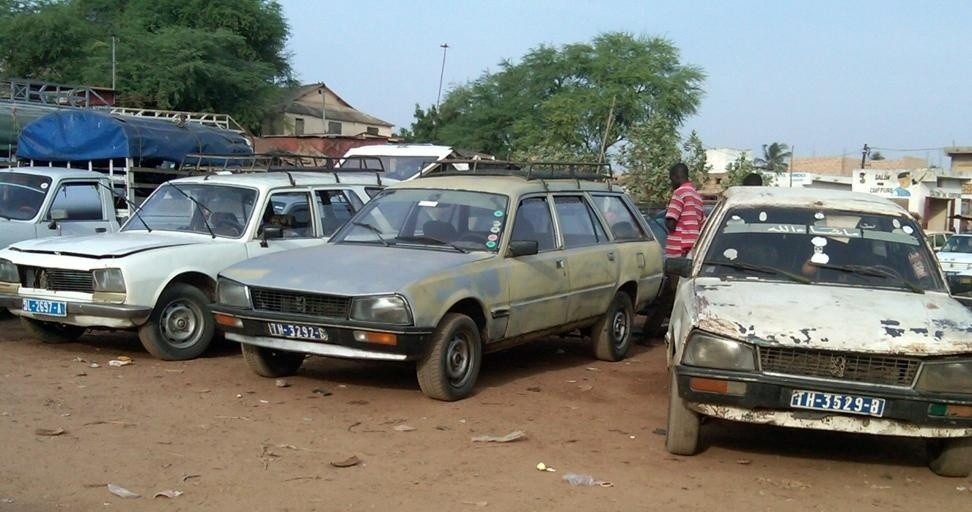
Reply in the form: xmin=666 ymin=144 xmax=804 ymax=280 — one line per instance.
xmin=436 ymin=42 xmax=448 ymax=113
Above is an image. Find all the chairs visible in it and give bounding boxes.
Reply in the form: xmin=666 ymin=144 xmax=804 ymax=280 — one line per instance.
xmin=499 ymin=219 xmax=535 ymax=241
xmin=612 ymin=222 xmax=638 ymax=240
xmin=211 ymin=212 xmax=238 ymax=229
xmin=270 ymin=216 xmax=296 ymax=227
xmin=738 ymin=243 xmax=780 ymax=274
xmin=422 ymin=220 xmax=456 ymax=241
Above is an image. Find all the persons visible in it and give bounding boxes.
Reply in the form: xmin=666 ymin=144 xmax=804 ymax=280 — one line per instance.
xmin=629 ymin=161 xmax=709 ymax=349
